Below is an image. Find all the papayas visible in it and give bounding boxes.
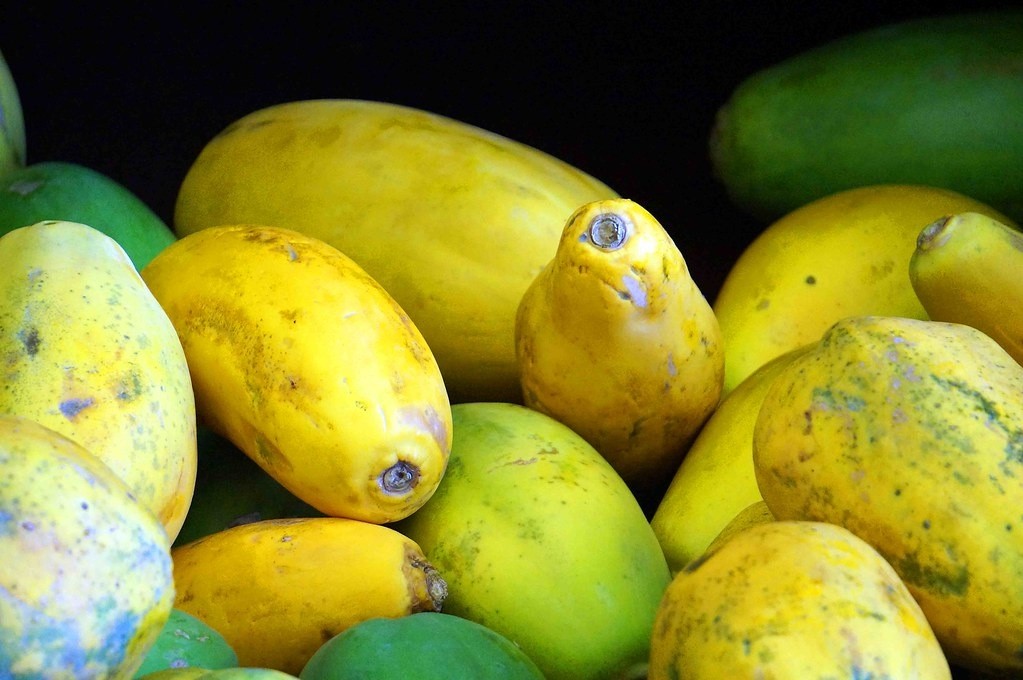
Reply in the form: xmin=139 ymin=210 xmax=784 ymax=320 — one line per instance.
xmin=0 ymin=7 xmax=1023 ymax=680
